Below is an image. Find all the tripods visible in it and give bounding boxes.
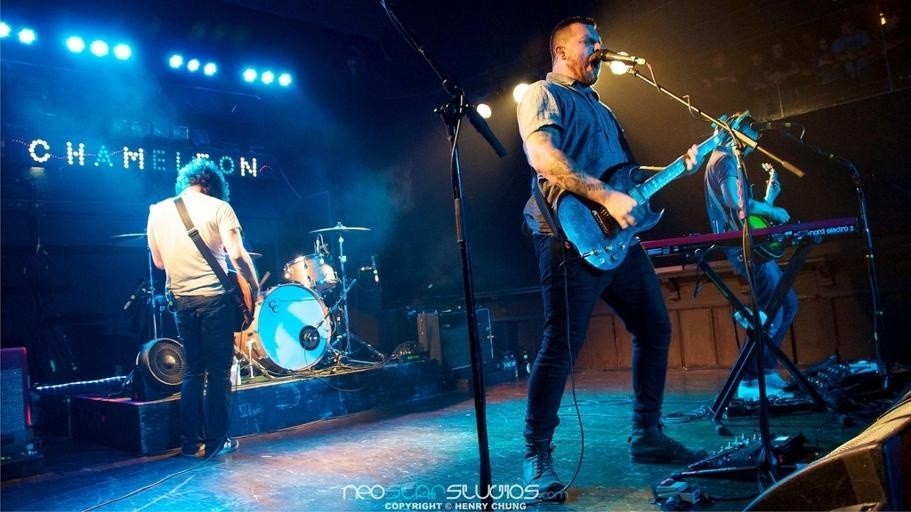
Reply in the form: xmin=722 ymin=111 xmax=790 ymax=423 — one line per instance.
xmin=329 ymin=264 xmax=385 ymax=364
xmin=670 ymin=162 xmax=814 ymax=488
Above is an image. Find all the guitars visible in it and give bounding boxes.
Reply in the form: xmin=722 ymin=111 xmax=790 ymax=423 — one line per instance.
xmin=229 ymin=271 xmax=255 ymax=331
xmin=745 ymin=163 xmax=786 ymax=262
xmin=558 ymin=110 xmax=751 ymax=271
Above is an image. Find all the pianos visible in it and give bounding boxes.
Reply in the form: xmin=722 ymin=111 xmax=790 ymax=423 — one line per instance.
xmin=631 ymin=215 xmax=859 ymax=256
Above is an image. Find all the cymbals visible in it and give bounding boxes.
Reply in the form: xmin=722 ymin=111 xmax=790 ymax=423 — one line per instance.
xmin=108 ymin=233 xmax=151 ymax=243
xmin=246 ymin=250 xmax=262 ymax=258
xmin=307 ymin=222 xmax=373 ymax=233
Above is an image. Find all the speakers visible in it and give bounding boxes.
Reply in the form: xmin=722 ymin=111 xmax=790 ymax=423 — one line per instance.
xmin=128 ymin=332 xmax=191 ymax=401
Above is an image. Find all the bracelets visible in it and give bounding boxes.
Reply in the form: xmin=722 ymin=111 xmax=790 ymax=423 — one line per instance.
xmin=252 ymin=287 xmax=258 ymax=291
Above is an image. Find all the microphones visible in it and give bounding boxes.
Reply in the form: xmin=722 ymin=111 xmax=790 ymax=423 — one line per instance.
xmin=748 ymin=117 xmax=795 ymax=133
xmin=594 ymin=48 xmax=641 ymax=64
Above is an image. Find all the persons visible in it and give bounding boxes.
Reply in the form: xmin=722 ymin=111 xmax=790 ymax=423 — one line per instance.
xmin=517 ymin=16 xmax=709 ymax=502
xmin=147 ymin=157 xmax=260 ymax=458
xmin=704 ymin=113 xmax=799 ymax=402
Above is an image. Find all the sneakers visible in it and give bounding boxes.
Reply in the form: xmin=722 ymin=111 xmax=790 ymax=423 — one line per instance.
xmin=205 ymin=438 xmax=240 ymax=457
xmin=750 ymin=368 xmax=792 ymax=388
xmin=629 ymin=432 xmax=710 ymax=466
xmin=181 ymin=443 xmax=205 ymax=458
xmin=522 ymin=449 xmax=566 ymax=505
xmin=737 ymin=383 xmax=794 ymax=401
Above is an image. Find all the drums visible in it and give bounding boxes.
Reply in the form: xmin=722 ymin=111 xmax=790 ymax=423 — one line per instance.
xmin=232 ymin=284 xmax=331 ymax=376
xmin=282 ymin=254 xmax=335 ymax=289
